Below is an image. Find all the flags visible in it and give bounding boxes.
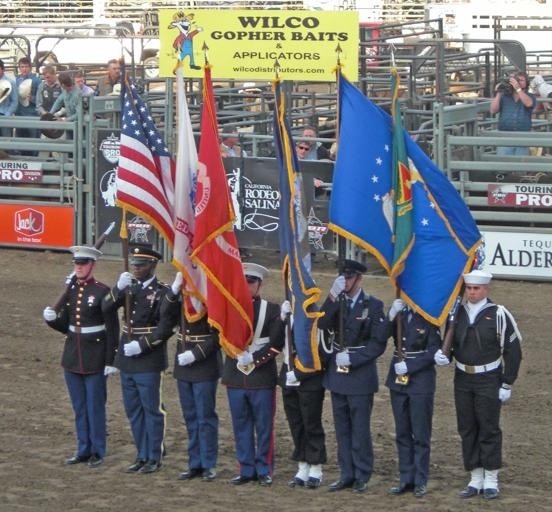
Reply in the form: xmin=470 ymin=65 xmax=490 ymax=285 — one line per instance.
xmin=190 ymin=60 xmax=257 ymax=360
xmin=172 ymin=62 xmax=210 ymax=325
xmin=271 ymin=75 xmax=326 ymax=375
xmin=391 ymin=69 xmax=416 ymax=289
xmin=115 ymin=69 xmax=176 ymax=252
xmin=328 ymin=69 xmax=482 ymax=326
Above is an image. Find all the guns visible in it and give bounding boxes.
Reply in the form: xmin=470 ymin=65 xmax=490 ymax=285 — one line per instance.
xmin=53 ymin=222 xmax=117 ymax=315
xmin=440 ymin=285 xmax=464 ymax=364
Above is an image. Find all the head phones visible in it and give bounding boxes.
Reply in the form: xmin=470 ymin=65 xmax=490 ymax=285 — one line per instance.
xmin=518 ymin=72 xmax=530 ymax=86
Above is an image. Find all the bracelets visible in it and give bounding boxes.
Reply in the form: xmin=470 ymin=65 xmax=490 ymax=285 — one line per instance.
xmin=516 ymin=88 xmax=522 ymax=93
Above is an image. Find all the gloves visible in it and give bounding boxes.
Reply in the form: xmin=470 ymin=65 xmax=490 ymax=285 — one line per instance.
xmin=498 ymin=388 xmax=511 ymax=402
xmin=388 ymin=298 xmax=405 ymax=322
xmin=177 ymin=350 xmax=196 ymax=366
xmin=394 ymin=360 xmax=408 ymax=375
xmin=123 ymin=340 xmax=142 ymax=357
xmin=117 ymin=272 xmax=133 ymax=290
xmin=330 ymin=275 xmax=346 ymax=298
xmin=434 ymin=349 xmax=450 ymax=366
xmin=335 ymin=352 xmax=351 ymax=366
xmin=285 ymin=370 xmax=300 ymax=386
xmin=43 ymin=306 xmax=57 ymax=321
xmin=237 ymin=351 xmax=254 ymax=367
xmin=392 ymin=350 xmax=425 ymax=359
xmin=103 ymin=365 xmax=117 ymax=376
xmin=280 ymin=300 xmax=293 ymax=321
xmin=171 ymin=272 xmax=183 ymax=295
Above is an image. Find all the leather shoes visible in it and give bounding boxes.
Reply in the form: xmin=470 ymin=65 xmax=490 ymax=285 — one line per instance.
xmin=305 ymin=477 xmax=320 ymax=488
xmin=126 ymin=459 xmax=147 ymax=473
xmin=413 ymin=485 xmax=426 ymax=496
xmin=258 ymin=474 xmax=273 ymax=486
xmin=353 ymin=480 xmax=368 ymax=493
xmin=231 ymin=475 xmax=248 ymax=484
xmin=88 ymin=453 xmax=103 ymax=467
xmin=177 ymin=468 xmax=200 ymax=479
xmin=138 ymin=460 xmax=163 ymax=475
xmin=460 ymin=486 xmax=484 ymax=497
xmin=288 ymin=477 xmax=304 ymax=487
xmin=201 ymin=468 xmax=218 ymax=481
xmin=389 ymin=483 xmax=412 ymax=495
xmin=327 ymin=480 xmax=347 ymax=491
xmin=484 ymin=488 xmax=500 ymax=499
xmin=65 ymin=456 xmax=87 ymax=465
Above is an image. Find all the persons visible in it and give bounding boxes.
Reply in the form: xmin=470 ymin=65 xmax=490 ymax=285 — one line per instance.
xmin=295 ymin=127 xmax=331 ymax=160
xmin=100 ymin=248 xmax=181 ymax=475
xmin=529 ymin=76 xmax=551 ymax=154
xmin=220 ymin=125 xmax=248 ymax=158
xmin=218 ymin=261 xmax=285 ymax=489
xmin=160 ymin=259 xmax=224 ymax=484
xmin=42 ymin=245 xmax=120 ymax=468
xmin=0 ymin=58 xmax=95 ymax=159
xmin=492 ymin=72 xmax=536 ymax=182
xmin=272 ymin=290 xmax=330 ymax=490
xmin=98 ymin=59 xmax=120 ymax=96
xmin=377 ymin=290 xmax=441 ymax=500
xmin=238 ymin=82 xmax=269 ymax=112
xmin=316 ymin=257 xmax=389 ymax=496
xmin=434 ymin=269 xmax=522 ymax=498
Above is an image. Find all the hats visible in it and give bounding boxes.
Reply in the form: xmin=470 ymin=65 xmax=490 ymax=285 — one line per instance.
xmin=335 ymin=259 xmax=367 ymax=279
xmin=67 ymin=245 xmax=103 ymax=264
xmin=462 ymin=270 xmax=493 ymax=285
xmin=0 ymin=79 xmax=12 ymax=103
xmin=129 ymin=246 xmax=163 ymax=265
xmin=38 ymin=113 xmax=65 ymax=138
xmin=17 ymin=79 xmax=32 ymax=107
xmin=241 ymin=262 xmax=269 ymax=280
xmin=106 ymin=84 xmax=121 ymax=95
xmin=237 ymin=82 xmax=262 ymax=94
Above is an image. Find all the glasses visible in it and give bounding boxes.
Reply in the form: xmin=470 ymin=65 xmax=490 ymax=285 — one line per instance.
xmin=299 ymin=146 xmax=309 ymax=151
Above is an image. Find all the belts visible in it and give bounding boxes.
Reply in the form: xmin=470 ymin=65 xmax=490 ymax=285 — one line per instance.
xmin=456 ymin=357 xmax=501 ymax=374
xmin=333 ymin=342 xmax=364 ymax=354
xmin=69 ymin=325 xmax=104 ymax=334
xmin=176 ymin=334 xmax=210 ymax=343
xmin=122 ymin=326 xmax=155 ymax=334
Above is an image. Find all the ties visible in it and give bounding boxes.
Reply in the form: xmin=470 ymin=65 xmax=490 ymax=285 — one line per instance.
xmin=346 ymin=299 xmax=353 ymax=315
xmin=138 ymin=283 xmax=143 ymax=289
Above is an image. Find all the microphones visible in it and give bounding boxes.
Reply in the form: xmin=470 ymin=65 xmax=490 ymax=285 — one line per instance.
xmin=522 ymin=86 xmax=528 ymax=90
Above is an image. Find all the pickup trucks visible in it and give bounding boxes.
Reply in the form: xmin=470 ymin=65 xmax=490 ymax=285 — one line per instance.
xmin=29 ymin=19 xmax=161 ymax=81
xmin=358 ymin=23 xmax=465 ymax=87
